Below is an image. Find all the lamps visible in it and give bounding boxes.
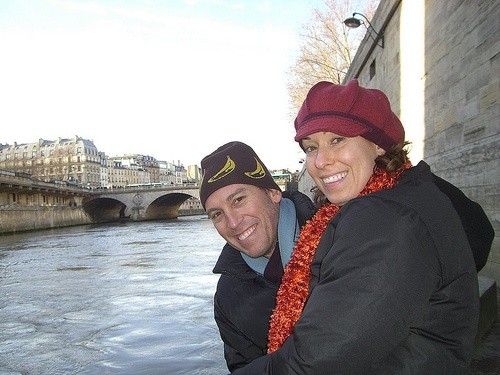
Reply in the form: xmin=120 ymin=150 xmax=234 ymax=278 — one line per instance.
xmin=344 ymin=13 xmax=384 ymax=49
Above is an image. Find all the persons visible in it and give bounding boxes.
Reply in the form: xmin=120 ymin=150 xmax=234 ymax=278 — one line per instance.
xmin=231 ymin=79 xmax=496 ymax=375
xmin=199 ymin=141 xmax=318 ymax=374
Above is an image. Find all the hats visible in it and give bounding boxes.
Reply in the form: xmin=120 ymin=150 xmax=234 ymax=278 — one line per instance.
xmin=294 ymin=79 xmax=406 ymax=152
xmin=199 ymin=141 xmax=282 ymax=213
